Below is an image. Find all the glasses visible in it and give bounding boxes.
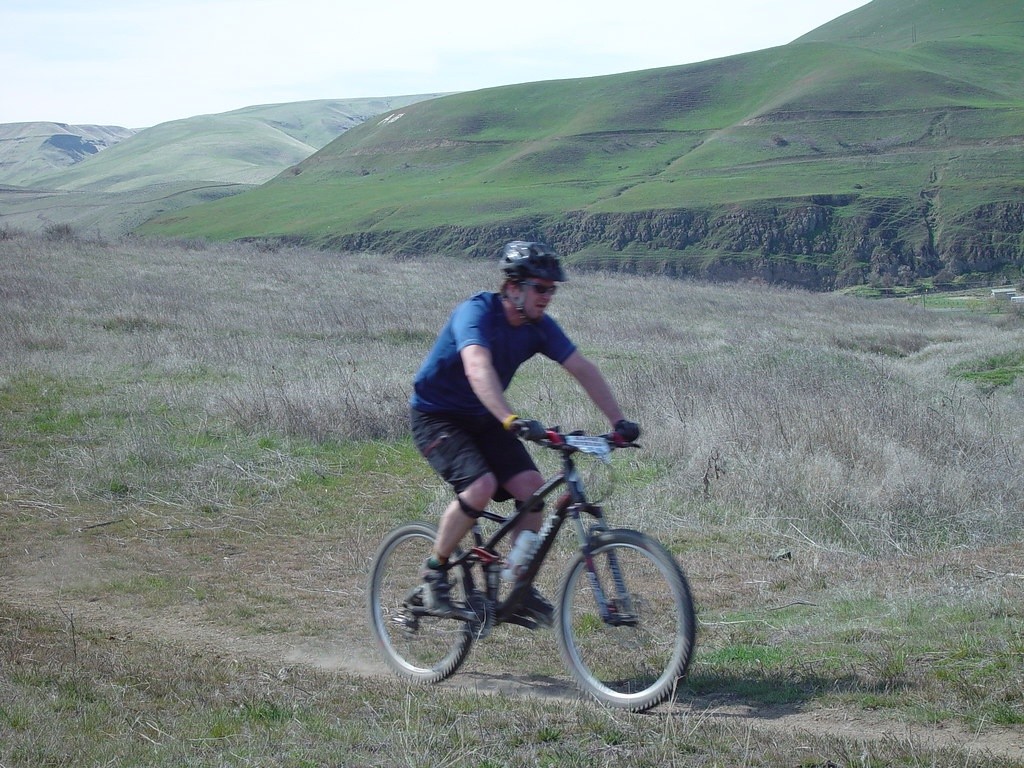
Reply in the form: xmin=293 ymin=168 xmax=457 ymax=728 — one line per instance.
xmin=520 ymin=283 xmax=558 ymax=294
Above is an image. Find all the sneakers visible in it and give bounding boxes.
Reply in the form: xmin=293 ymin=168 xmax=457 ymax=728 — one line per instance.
xmin=419 ymin=559 xmax=453 ymax=615
xmin=525 ymin=587 xmax=555 ymax=615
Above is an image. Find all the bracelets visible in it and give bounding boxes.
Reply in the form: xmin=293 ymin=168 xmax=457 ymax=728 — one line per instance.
xmin=503 ymin=414 xmax=519 ymax=431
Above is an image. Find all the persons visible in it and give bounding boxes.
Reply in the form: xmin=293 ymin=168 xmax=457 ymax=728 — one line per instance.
xmin=410 ymin=241 xmax=641 ymax=617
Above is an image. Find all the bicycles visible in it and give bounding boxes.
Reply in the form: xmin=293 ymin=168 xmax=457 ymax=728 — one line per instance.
xmin=367 ymin=424 xmax=695 ymax=713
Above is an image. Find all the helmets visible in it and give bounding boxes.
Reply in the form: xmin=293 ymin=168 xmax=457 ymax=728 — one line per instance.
xmin=498 ymin=240 xmax=568 ymax=282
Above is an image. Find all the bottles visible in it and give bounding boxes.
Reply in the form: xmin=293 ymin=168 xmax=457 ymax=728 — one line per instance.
xmin=498 ymin=529 xmax=539 ymax=584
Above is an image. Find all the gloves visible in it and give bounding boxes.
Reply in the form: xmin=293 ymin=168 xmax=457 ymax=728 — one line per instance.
xmin=504 ymin=415 xmax=545 ymax=441
xmin=615 ymin=421 xmax=639 ymax=443
